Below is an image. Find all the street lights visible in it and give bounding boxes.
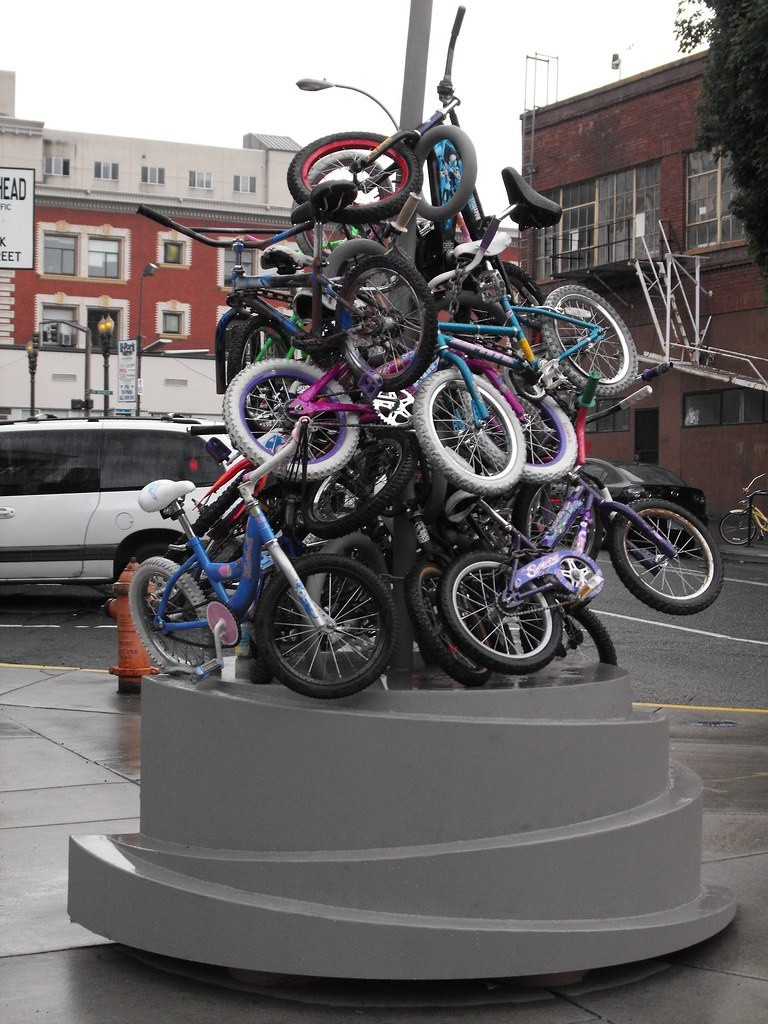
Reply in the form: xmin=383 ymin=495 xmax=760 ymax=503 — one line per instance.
xmin=137 ymin=261 xmax=172 ymax=421
xmin=98 ymin=315 xmax=114 ymax=415
xmin=297 ymin=79 xmax=399 ymax=136
xmin=26 ymin=340 xmax=37 ymax=414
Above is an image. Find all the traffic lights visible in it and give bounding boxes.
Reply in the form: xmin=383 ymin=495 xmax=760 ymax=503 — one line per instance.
xmin=52 ymin=322 xmax=62 ymax=342
xmin=32 ymin=331 xmax=42 ymax=350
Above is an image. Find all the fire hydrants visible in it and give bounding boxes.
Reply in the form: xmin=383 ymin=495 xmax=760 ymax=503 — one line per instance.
xmin=105 ymin=561 xmax=157 ymax=696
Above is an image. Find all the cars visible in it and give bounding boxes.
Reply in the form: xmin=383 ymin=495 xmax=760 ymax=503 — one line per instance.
xmin=0 ymin=414 xmax=227 ymax=608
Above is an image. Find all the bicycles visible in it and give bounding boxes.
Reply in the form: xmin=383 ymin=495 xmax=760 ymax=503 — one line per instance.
xmin=721 ymin=473 xmax=767 ymax=546
xmin=127 ymin=6 xmax=723 ymax=702
